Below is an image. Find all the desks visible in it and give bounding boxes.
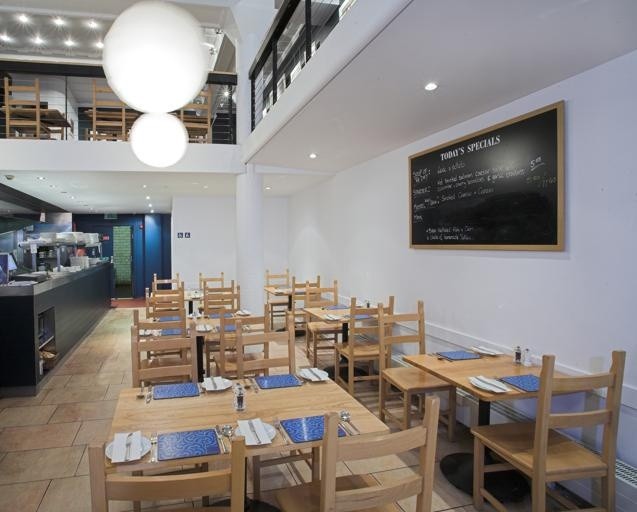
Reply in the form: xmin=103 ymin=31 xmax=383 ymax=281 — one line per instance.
xmin=0 ymin=106 xmax=72 ymax=140
xmin=88 ymin=109 xmax=214 ymax=144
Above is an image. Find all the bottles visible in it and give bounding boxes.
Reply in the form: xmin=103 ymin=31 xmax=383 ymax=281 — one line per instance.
xmin=36 ymin=250 xmax=55 ymax=271
xmin=513 ymin=345 xmax=533 ymax=366
xmin=366 ymin=301 xmax=369 ymax=308
xmin=233 ymin=382 xmax=247 ymax=411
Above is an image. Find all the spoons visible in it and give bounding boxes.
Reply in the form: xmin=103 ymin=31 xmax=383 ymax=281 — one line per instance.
xmin=221 ymin=425 xmax=232 ymax=445
xmin=136 ymin=381 xmax=145 ymax=399
xmin=340 ymin=411 xmax=359 ymax=434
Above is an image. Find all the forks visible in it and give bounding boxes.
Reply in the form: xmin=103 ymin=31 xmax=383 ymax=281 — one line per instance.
xmin=149 ymin=433 xmax=157 ymax=464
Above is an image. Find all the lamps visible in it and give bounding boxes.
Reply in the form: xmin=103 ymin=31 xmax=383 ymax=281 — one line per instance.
xmin=129 ymin=112 xmax=190 ymax=168
xmin=100 ymin=0 xmax=212 ymax=112
xmin=215 ymin=28 xmax=222 ymax=34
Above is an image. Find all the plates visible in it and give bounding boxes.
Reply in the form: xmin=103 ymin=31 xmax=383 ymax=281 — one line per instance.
xmin=38 ymin=232 xmax=98 ymax=245
xmin=471 ymin=381 xmax=505 ymax=389
xmin=299 ymin=369 xmax=328 ymax=382
xmin=200 ymin=378 xmax=231 ymax=392
xmin=48 ymin=256 xmax=108 ymax=279
xmin=103 ymin=436 xmax=151 ymax=459
xmin=234 ymin=422 xmax=276 ymax=442
xmin=135 ymin=309 xmax=251 ymax=337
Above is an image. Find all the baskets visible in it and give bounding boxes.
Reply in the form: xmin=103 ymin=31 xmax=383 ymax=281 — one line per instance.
xmin=39 ymin=350 xmax=60 ymax=369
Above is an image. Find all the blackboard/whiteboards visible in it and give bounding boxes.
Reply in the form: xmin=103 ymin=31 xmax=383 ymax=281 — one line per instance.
xmin=408 ymin=100 xmax=564 ymax=250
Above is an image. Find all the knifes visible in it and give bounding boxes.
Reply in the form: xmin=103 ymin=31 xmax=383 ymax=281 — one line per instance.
xmin=306 ymin=367 xmax=320 ymax=379
xmin=214 ymin=424 xmax=229 ymax=454
xmin=247 ymin=420 xmax=260 ymax=443
xmin=210 ymin=376 xmax=216 ymax=389
xmin=125 ymin=432 xmax=131 ymax=461
xmin=144 ymin=384 xmax=152 ymax=403
xmin=248 ymin=376 xmax=258 ymax=393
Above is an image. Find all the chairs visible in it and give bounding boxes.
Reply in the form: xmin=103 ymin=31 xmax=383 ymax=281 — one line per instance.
xmin=180 ymin=84 xmax=213 ymax=144
xmin=3 ymin=76 xmax=51 ymax=139
xmin=92 ymin=80 xmax=126 ymax=141
